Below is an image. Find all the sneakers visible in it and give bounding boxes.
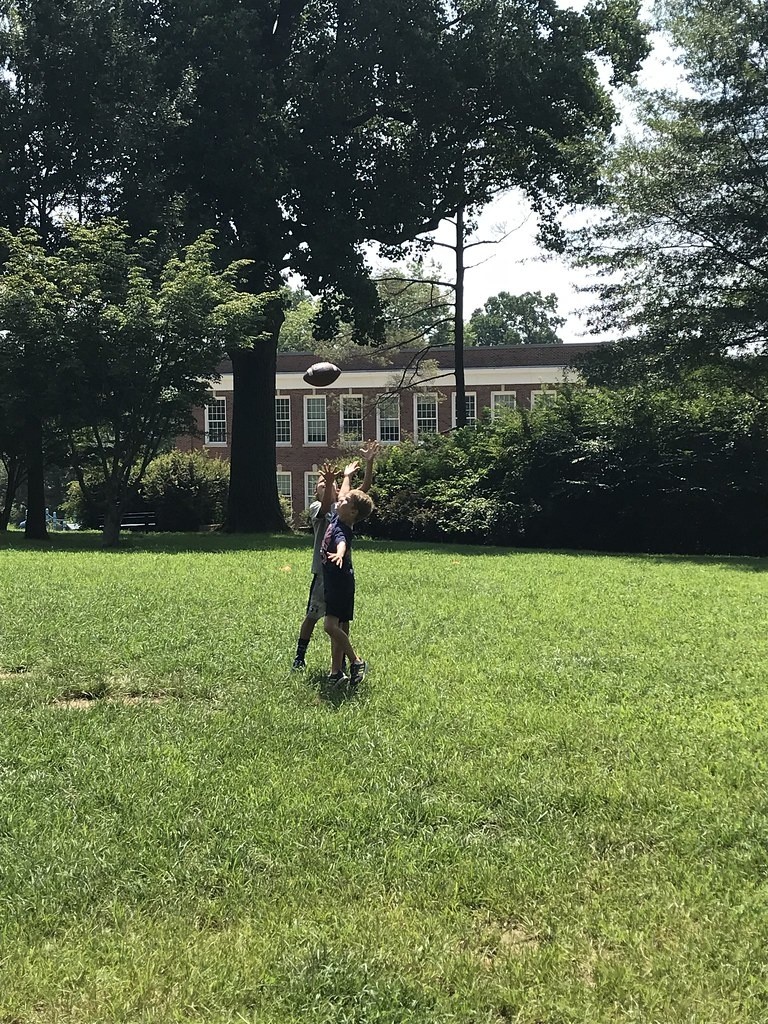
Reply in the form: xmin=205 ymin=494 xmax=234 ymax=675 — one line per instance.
xmin=350 ymin=658 xmax=367 ymax=686
xmin=328 ymin=669 xmax=349 ymax=691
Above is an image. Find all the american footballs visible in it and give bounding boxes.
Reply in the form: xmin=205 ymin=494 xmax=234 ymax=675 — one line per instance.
xmin=303 ymin=361 xmax=342 ymax=387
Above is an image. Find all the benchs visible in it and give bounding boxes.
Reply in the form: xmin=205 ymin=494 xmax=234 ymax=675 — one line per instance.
xmin=97 ymin=511 xmax=155 ymax=534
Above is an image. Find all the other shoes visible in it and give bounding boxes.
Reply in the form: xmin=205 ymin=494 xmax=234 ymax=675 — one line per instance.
xmin=342 ymin=665 xmax=347 ymax=674
xmin=294 ymin=658 xmax=306 ymax=671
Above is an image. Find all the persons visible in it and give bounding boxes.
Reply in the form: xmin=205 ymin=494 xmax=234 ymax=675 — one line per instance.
xmin=320 ymin=460 xmax=373 ymax=690
xmin=292 ymin=438 xmax=379 ymax=672
